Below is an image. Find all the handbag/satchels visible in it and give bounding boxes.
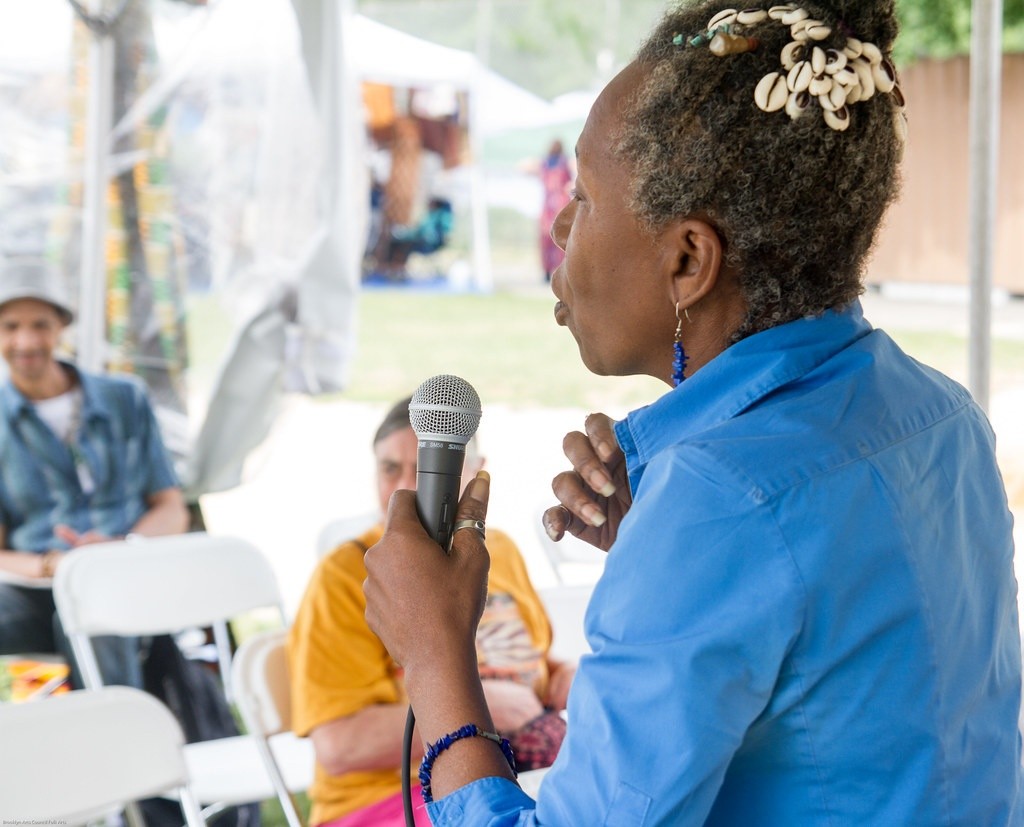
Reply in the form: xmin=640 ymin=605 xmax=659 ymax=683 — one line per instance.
xmin=501 ymin=708 xmax=566 ymax=774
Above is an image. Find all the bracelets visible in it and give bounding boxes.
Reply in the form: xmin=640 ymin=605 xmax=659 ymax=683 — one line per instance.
xmin=418 ymin=724 xmax=517 ymax=803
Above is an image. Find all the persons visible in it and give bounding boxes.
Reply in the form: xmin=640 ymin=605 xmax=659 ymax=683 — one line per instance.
xmin=389 ymin=197 xmax=452 ymax=281
xmin=522 ymin=137 xmax=576 ymax=285
xmin=287 ymin=395 xmax=577 ymax=827
xmin=0 ymin=255 xmax=190 ymax=690
xmin=362 ymin=0 xmax=1024 ymax=827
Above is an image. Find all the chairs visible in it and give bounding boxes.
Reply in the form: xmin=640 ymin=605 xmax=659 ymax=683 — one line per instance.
xmin=0 ymin=531 xmax=383 ymax=827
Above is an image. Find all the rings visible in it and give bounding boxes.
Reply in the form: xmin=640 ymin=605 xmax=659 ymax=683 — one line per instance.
xmin=560 ymin=506 xmax=571 ymax=529
xmin=454 ymin=519 xmax=486 ymax=539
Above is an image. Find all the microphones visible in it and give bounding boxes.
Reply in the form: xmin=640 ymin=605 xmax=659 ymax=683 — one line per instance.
xmin=409 ymin=374 xmax=483 ymax=553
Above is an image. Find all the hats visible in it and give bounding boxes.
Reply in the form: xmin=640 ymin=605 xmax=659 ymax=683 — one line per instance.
xmin=0 ymin=257 xmax=73 ymax=326
